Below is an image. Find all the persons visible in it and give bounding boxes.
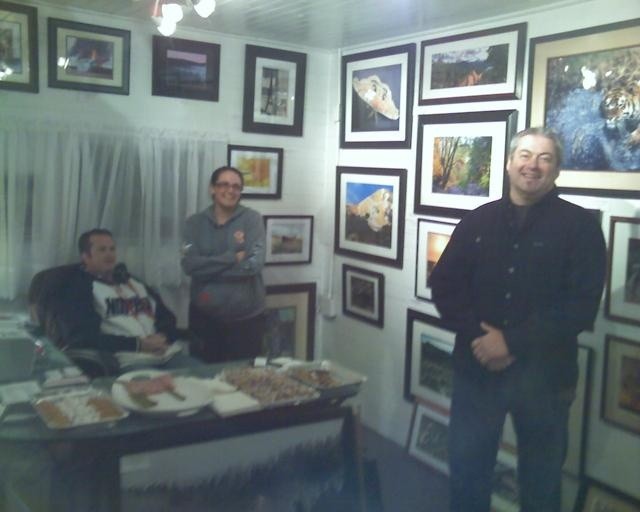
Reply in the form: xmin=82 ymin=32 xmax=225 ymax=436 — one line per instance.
xmin=427 ymin=127 xmax=610 ymax=511
xmin=181 ymin=164 xmax=268 ymax=362
xmin=48 ymin=227 xmax=184 ymax=375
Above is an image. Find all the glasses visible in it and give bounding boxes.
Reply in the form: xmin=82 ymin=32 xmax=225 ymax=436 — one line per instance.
xmin=215 ymin=182 xmax=243 ymax=192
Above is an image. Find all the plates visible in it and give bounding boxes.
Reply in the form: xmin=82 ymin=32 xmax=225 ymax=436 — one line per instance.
xmin=281 ymin=359 xmax=366 ymax=393
xmin=32 ymin=390 xmax=129 ymax=432
xmin=215 ymin=369 xmax=321 ymax=419
xmin=113 ymin=371 xmax=213 ymax=417
